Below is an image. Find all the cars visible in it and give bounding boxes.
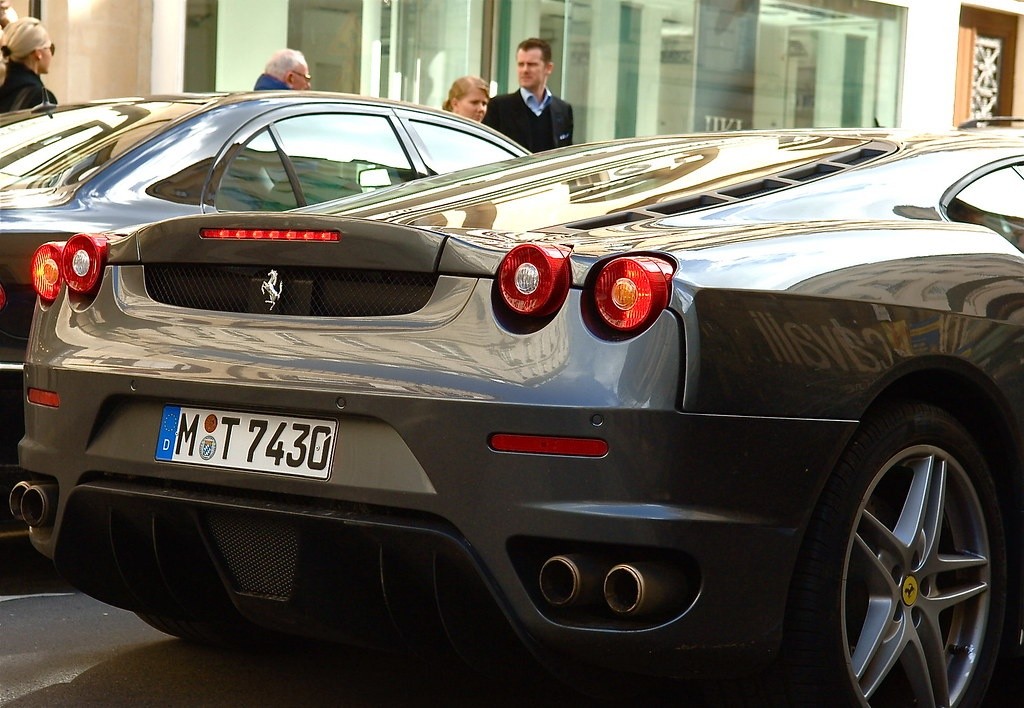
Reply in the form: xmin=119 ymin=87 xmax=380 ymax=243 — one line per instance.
xmin=0 ymin=87 xmax=532 ymax=472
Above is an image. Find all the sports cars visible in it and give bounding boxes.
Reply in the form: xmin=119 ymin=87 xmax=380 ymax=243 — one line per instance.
xmin=5 ymin=113 xmax=1024 ymax=707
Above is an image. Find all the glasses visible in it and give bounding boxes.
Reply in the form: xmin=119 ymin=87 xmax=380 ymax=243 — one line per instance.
xmin=44 ymin=44 xmax=55 ymax=56
xmin=293 ymin=69 xmax=312 ymax=82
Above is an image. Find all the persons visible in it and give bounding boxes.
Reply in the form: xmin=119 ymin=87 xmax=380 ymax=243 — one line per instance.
xmin=443 ymin=76 xmax=489 ymax=123
xmin=0 ymin=17 xmax=58 ymax=113
xmin=481 ymin=38 xmax=574 ymax=154
xmin=253 ymin=49 xmax=310 ymax=92
